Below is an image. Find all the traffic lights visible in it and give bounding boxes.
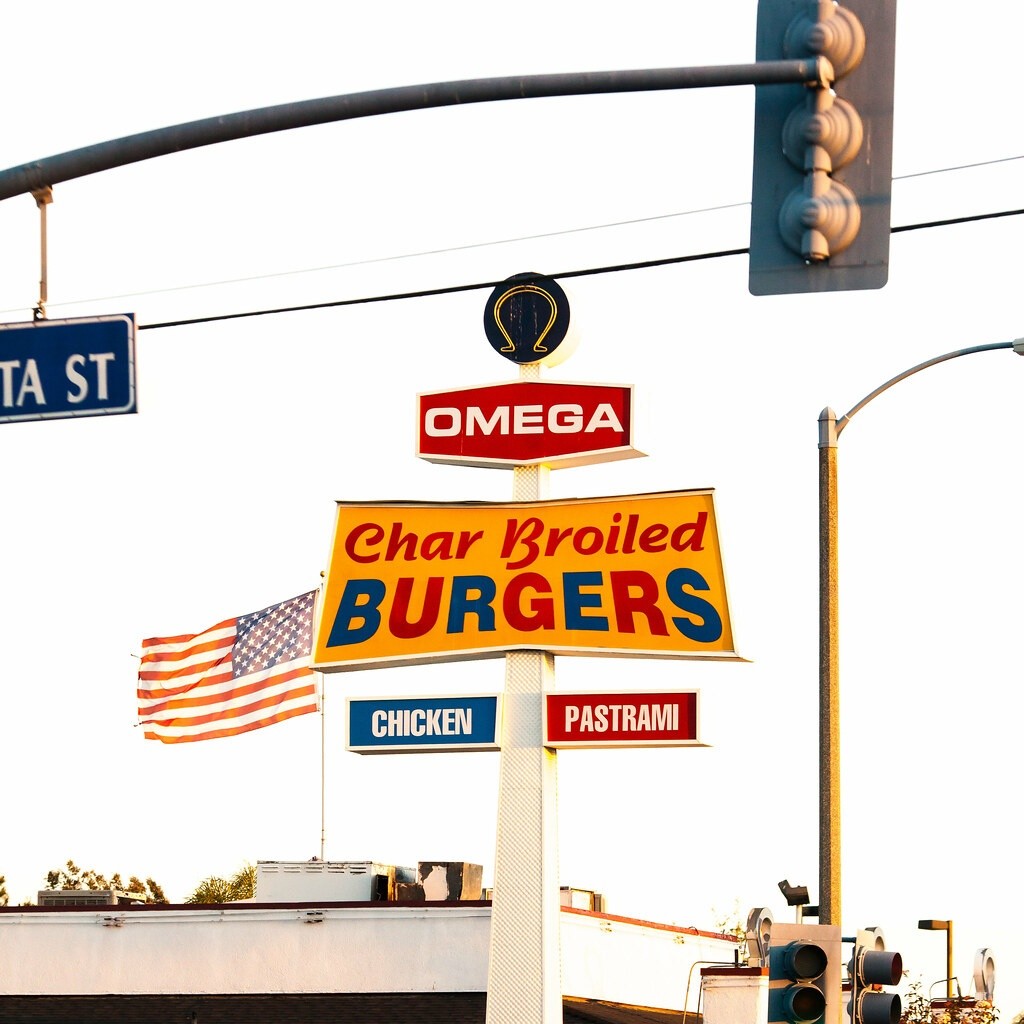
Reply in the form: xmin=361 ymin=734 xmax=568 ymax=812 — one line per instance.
xmin=767 ymin=921 xmax=844 ymax=1024
xmin=853 ymin=926 xmax=906 ymax=1024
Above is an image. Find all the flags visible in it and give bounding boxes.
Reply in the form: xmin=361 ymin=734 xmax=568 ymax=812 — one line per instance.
xmin=137 ymin=589 xmax=319 ymax=744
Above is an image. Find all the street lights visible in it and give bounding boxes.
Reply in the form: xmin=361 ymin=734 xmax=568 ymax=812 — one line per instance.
xmin=918 ymin=919 xmax=954 ymax=1001
xmin=812 ymin=336 xmax=1024 ymax=923
